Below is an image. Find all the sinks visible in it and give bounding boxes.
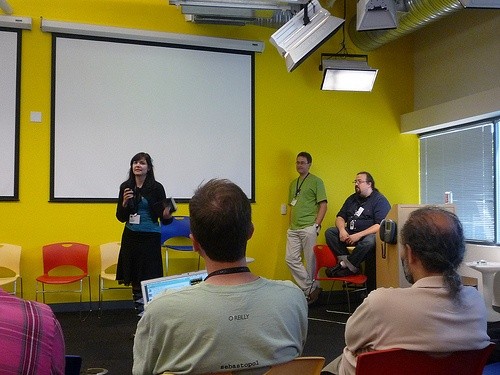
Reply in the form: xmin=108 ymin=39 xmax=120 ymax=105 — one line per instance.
xmin=466 ymin=261 xmax=500 ymax=273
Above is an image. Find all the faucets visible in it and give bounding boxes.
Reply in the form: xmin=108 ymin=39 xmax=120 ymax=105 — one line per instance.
xmin=477 ymin=260 xmax=486 ymax=264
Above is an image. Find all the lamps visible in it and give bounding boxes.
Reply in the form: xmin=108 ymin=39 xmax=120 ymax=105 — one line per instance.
xmin=269 ymin=0 xmax=409 ymax=91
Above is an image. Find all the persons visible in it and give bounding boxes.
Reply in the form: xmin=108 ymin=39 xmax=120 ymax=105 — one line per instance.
xmin=324 ymin=203 xmax=492 ymax=375
xmin=117 ymin=152 xmax=175 ymax=340
xmin=325 ymin=172 xmax=392 ymax=278
xmin=131 ymin=178 xmax=308 ymax=375
xmin=0 ymin=286 xmax=66 ymax=375
xmin=285 ymin=152 xmax=322 ymax=307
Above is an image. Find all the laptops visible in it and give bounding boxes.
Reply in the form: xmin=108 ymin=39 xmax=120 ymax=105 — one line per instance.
xmin=141 ymin=269 xmax=209 ymax=306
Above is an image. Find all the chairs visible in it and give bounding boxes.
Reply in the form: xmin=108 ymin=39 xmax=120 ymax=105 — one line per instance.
xmin=158 ymin=216 xmax=200 ymax=277
xmin=36 ymin=242 xmax=93 ymax=322
xmin=308 ymin=245 xmax=368 ymax=325
xmin=0 ymin=244 xmax=23 ymax=298
xmin=97 ymin=242 xmax=132 ymax=319
xmin=167 ymin=345 xmax=500 ymax=375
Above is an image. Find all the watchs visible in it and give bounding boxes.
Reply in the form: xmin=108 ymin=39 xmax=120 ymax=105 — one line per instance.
xmin=316 ymin=224 xmax=320 ymax=228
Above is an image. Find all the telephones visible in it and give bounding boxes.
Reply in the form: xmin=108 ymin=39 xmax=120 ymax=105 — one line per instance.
xmin=380 ymin=219 xmax=397 ymax=245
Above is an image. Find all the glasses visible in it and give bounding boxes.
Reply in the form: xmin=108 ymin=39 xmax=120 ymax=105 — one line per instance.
xmin=352 ymin=179 xmax=371 ymax=185
xmin=295 ymin=162 xmax=309 ymax=166
xmin=133 ymin=161 xmax=149 ymax=165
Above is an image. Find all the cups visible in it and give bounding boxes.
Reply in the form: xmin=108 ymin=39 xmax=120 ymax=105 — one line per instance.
xmin=445 ymin=192 xmax=453 ymax=205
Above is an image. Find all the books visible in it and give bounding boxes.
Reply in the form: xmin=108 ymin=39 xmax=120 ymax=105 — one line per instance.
xmin=153 ymin=197 xmax=178 ymax=216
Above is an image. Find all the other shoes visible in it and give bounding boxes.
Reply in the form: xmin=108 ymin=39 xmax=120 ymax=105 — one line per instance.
xmin=325 ymin=259 xmax=359 ymax=278
xmin=305 ymin=286 xmax=323 ymax=305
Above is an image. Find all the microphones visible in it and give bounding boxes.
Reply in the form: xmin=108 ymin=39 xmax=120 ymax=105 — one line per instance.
xmin=128 ymin=199 xmax=134 ymax=210
